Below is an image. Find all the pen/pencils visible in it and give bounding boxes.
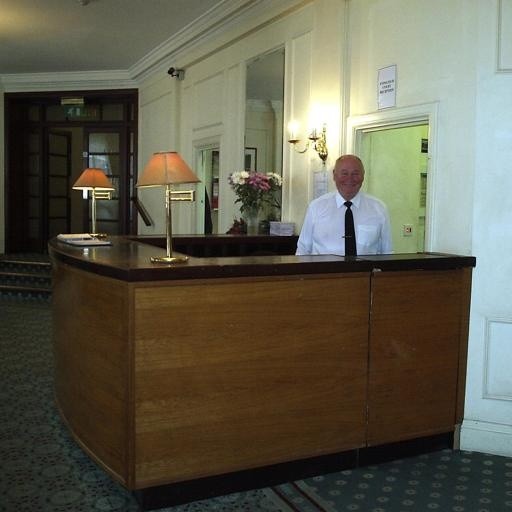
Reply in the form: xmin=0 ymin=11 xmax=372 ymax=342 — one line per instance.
xmin=63 ymin=237 xmax=97 ymax=241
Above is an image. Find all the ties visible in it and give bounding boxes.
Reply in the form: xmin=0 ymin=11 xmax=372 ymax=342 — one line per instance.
xmin=344 ymin=201 xmax=357 ymax=256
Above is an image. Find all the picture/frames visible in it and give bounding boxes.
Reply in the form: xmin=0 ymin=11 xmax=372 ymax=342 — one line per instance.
xmin=243 ymin=146 xmax=258 ymax=173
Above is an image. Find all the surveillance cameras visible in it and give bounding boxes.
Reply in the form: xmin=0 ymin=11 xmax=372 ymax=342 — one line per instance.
xmin=168 ymin=67 xmax=175 ymax=75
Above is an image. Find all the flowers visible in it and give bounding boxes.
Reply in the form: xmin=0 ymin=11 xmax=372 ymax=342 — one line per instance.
xmin=227 ymin=170 xmax=283 ymax=216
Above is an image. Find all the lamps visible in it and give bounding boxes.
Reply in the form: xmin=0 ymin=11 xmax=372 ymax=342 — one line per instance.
xmin=72 ymin=168 xmax=116 ymax=237
xmin=133 ymin=151 xmax=202 ymax=264
xmin=286 ymin=119 xmax=329 ymax=164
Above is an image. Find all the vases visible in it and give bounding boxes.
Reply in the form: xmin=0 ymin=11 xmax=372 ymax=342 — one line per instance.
xmin=243 ymin=206 xmax=261 ymax=235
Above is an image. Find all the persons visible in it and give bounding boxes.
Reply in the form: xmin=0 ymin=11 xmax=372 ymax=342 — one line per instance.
xmin=295 ymin=154 xmax=391 ymax=255
